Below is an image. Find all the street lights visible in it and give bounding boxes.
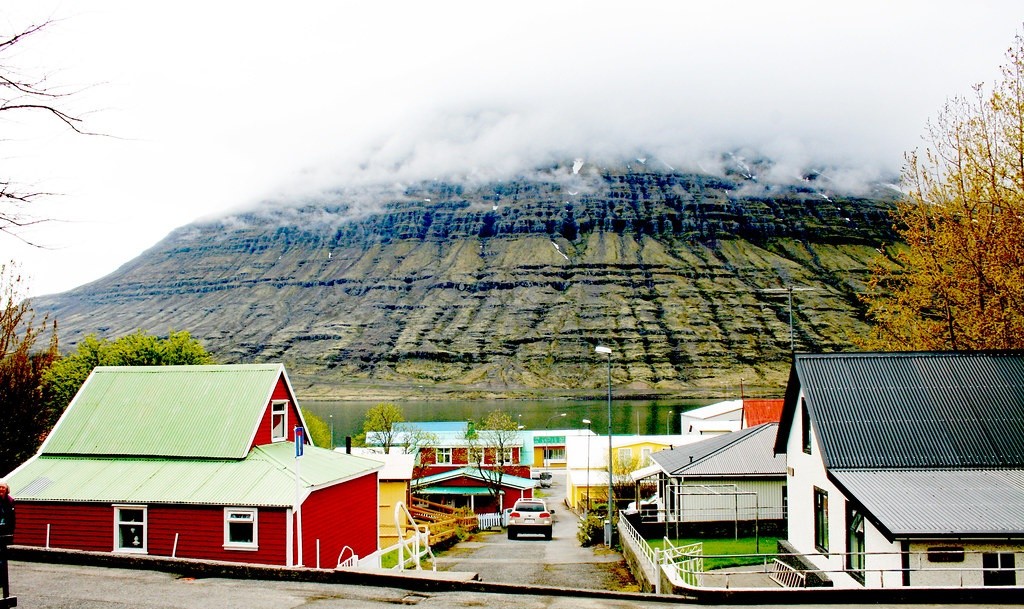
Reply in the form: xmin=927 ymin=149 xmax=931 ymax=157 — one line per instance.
xmin=544 ymin=413 xmax=567 ymax=475
xmin=594 ymin=346 xmax=615 ymax=541
xmin=667 ymin=410 xmax=673 ymax=438
xmin=581 ymin=418 xmax=592 ymax=511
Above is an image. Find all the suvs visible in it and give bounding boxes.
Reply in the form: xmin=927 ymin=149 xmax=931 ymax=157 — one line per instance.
xmin=505 ymin=498 xmax=555 ymax=541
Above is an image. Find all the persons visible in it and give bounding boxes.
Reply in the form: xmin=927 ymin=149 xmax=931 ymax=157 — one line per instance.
xmin=0 ymin=483 xmax=16 ymax=535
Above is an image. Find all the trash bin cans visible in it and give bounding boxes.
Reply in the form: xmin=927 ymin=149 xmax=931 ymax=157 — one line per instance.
xmin=503 ymin=508 xmax=513 ymax=528
xmin=642 ymin=504 xmax=658 ymax=522
